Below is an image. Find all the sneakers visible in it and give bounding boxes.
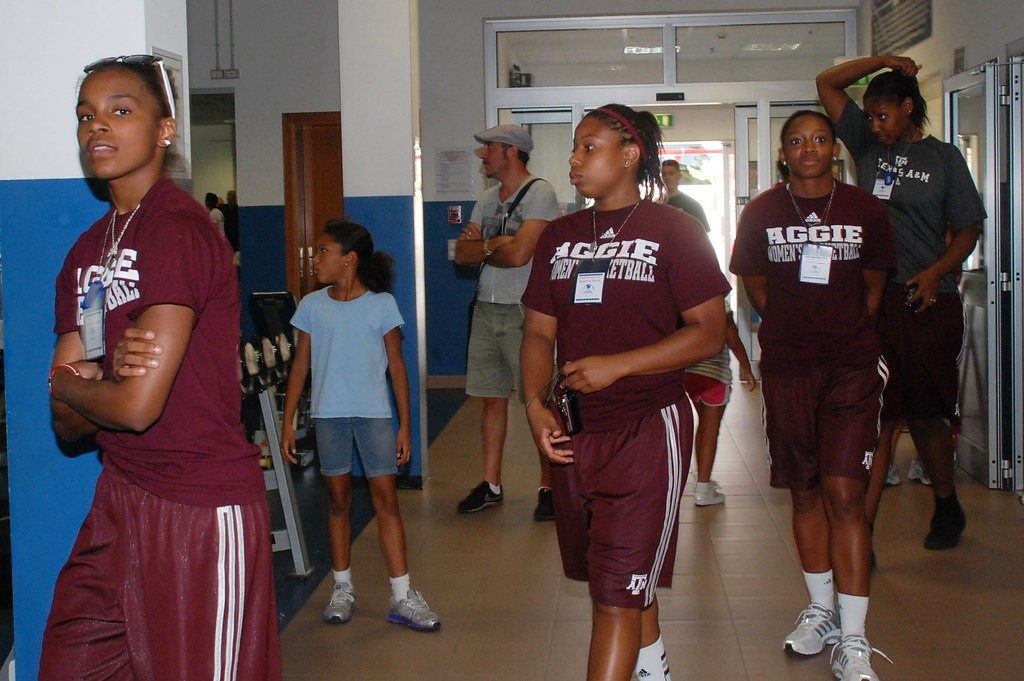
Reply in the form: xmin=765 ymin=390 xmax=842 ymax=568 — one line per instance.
xmin=457 ymin=480 xmax=504 ymax=513
xmin=386 ymin=587 xmax=442 ymax=631
xmin=879 ymin=465 xmax=901 ymax=487
xmin=908 ymin=459 xmax=932 ymax=486
xmin=782 ymin=600 xmax=841 ymax=656
xmin=533 ymin=487 xmax=554 ymax=520
xmin=832 ymin=634 xmax=879 ymax=681
xmin=924 ymin=487 xmax=967 ymax=550
xmin=693 ymin=482 xmax=726 ymax=506
xmin=324 ymin=581 xmax=356 ymax=624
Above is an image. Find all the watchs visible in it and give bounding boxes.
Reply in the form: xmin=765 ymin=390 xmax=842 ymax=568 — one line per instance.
xmin=484 ymin=239 xmax=492 ymax=256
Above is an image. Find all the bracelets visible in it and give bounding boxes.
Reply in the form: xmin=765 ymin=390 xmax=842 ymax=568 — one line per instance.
xmin=48 ymin=363 xmax=79 ymax=398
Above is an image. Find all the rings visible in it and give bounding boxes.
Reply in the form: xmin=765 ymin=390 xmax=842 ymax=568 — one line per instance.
xmin=929 ymin=298 xmax=937 ymax=303
xmin=468 ymin=232 xmax=473 ymax=236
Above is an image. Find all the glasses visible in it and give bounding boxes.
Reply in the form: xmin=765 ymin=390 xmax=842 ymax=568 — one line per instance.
xmin=85 ymin=53 xmax=176 ymax=123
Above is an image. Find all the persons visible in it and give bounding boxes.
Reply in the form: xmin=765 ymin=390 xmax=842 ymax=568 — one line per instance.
xmin=454 ymin=126 xmax=565 ymax=520
xmin=729 ymin=110 xmax=902 ymax=681
xmin=816 ymin=56 xmax=986 ymax=573
xmin=37 ymin=58 xmax=281 ymax=681
xmin=654 ymin=160 xmax=710 ymax=233
xmin=281 ymin=219 xmax=442 ymax=633
xmin=519 ymin=105 xmax=734 ymax=681
xmin=205 ymin=188 xmax=239 ymax=253
xmin=683 ymin=297 xmax=757 ymax=506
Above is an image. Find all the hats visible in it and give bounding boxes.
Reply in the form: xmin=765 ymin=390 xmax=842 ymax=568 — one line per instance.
xmin=474 ymin=123 xmax=534 ymax=153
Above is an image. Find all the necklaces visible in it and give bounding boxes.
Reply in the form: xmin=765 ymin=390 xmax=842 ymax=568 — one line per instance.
xmin=790 ymin=187 xmax=831 ymax=226
xmin=883 ymin=127 xmax=920 ymax=186
xmin=110 ymin=205 xmax=141 ymax=268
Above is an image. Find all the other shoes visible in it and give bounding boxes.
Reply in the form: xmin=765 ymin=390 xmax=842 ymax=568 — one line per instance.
xmin=953 ymin=452 xmax=961 ymax=470
